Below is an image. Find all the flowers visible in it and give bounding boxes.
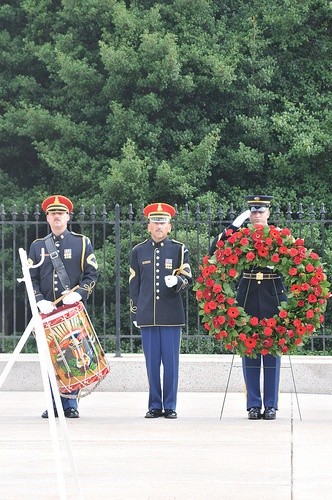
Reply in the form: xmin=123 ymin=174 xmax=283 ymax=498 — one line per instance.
xmin=192 ymin=222 xmax=332 ymax=357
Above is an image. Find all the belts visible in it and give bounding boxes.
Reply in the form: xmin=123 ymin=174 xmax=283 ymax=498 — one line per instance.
xmin=242 ymin=272 xmax=281 ymax=280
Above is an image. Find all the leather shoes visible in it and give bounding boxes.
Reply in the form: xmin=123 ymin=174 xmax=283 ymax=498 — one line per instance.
xmin=164 ymin=408 xmax=178 ymax=418
xmin=64 ymin=408 xmax=79 ymax=418
xmin=42 ymin=409 xmax=58 ymax=417
xmin=145 ymin=409 xmax=162 ymax=418
xmin=263 ymin=407 xmax=277 ymax=419
xmin=248 ymin=408 xmax=260 ymax=419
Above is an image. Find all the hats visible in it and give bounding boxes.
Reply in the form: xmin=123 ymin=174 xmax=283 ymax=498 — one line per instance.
xmin=41 ymin=195 xmax=73 ymax=214
xmin=243 ymin=194 xmax=274 ymax=212
xmin=143 ymin=202 xmax=175 ymax=223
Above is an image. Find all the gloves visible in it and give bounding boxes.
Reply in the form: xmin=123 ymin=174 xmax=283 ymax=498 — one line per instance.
xmin=233 ymin=209 xmax=251 ymax=227
xmin=37 ymin=300 xmax=57 ymax=314
xmin=133 ymin=320 xmax=140 ymax=329
xmin=165 ymin=275 xmax=177 ymax=287
xmin=62 ymin=291 xmax=83 ymax=305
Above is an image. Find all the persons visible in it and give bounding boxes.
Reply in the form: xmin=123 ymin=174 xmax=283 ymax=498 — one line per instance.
xmin=128 ymin=204 xmax=193 ymax=419
xmin=208 ymin=196 xmax=286 ymax=421
xmin=27 ymin=195 xmax=97 ymax=418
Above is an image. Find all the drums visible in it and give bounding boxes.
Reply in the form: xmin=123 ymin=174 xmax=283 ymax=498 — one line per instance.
xmin=31 ymin=302 xmax=112 ymax=393
